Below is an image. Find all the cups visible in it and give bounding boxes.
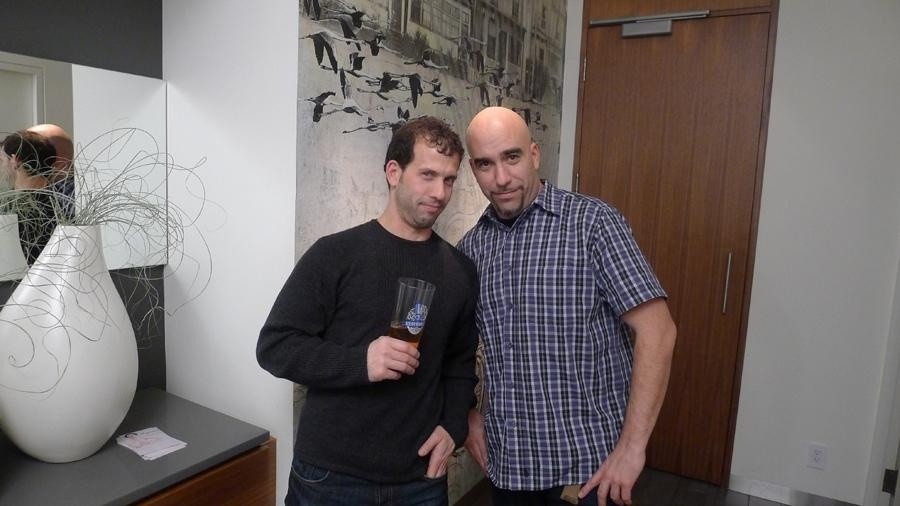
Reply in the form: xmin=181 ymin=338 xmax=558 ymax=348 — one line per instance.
xmin=383 ymin=275 xmax=438 ymax=347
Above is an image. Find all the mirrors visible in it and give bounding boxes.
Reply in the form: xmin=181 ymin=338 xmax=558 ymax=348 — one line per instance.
xmin=0 ymin=45 xmax=171 ymax=283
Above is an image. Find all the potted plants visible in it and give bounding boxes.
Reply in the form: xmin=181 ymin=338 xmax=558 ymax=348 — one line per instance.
xmin=0 ymin=122 xmax=215 ymax=465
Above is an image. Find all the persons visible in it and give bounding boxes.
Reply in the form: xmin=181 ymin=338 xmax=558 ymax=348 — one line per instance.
xmin=448 ymin=105 xmax=678 ymax=506
xmin=25 ymin=124 xmax=82 ymax=240
xmin=0 ymin=126 xmax=58 ymax=270
xmin=252 ymin=114 xmax=480 ymax=506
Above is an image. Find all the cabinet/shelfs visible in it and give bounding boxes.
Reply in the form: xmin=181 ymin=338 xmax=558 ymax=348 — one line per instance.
xmin=1 ymin=386 xmax=277 ymax=505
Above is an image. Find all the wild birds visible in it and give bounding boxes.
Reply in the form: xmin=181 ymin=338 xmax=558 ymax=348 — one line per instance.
xmin=298 ymin=0 xmax=549 ymax=136
